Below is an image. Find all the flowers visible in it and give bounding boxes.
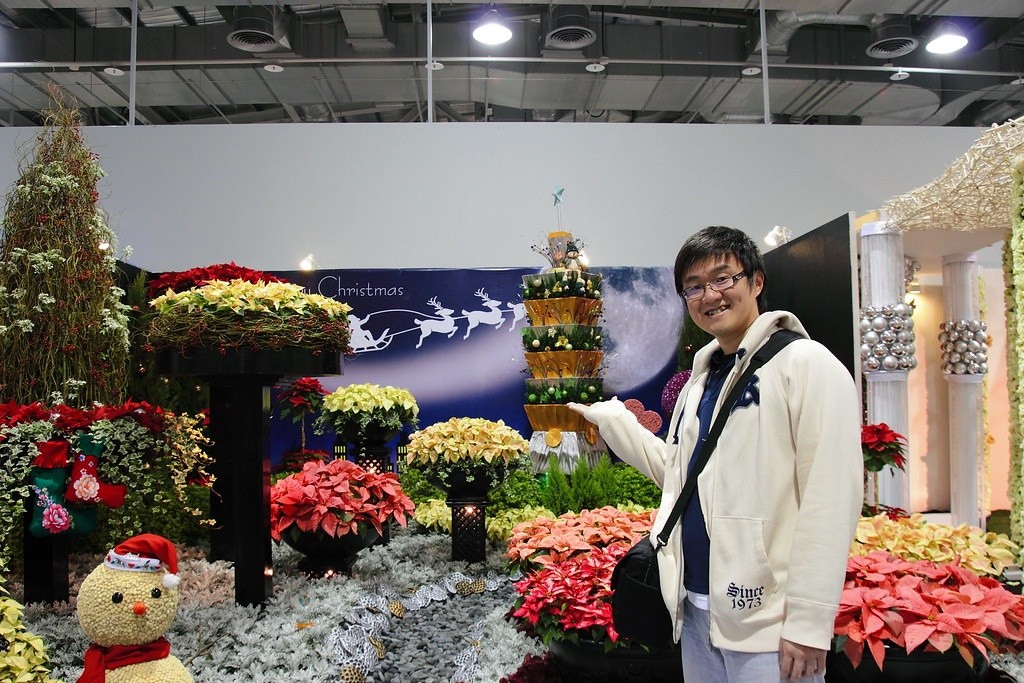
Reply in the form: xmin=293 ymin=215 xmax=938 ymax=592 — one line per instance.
xmin=0 ymin=77 xmax=1024 ymax=683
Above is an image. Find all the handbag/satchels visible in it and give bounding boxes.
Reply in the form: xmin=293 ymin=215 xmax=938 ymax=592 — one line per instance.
xmin=611 ymin=535 xmax=674 ymax=644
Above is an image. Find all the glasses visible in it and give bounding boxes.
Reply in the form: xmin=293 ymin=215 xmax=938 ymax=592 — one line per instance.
xmin=682 ymin=270 xmax=748 ymax=300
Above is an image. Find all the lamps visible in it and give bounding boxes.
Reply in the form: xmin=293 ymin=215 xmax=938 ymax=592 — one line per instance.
xmin=473 ymin=3 xmax=512 ymax=45
xmin=925 ymin=18 xmax=968 ymax=53
xmin=448 ymin=468 xmax=488 ymax=563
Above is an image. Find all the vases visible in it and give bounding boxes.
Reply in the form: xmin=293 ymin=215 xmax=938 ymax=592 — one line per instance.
xmin=155 ymin=345 xmax=234 ymax=565
xmin=171 ymin=344 xmax=345 ymax=610
xmin=858 ymin=643 xmax=989 ymax=683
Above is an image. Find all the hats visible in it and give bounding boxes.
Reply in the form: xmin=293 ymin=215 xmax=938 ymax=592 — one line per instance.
xmin=103 ymin=534 xmax=182 ymax=589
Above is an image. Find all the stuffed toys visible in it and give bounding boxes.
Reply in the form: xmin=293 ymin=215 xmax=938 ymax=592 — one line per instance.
xmin=76 ymin=534 xmax=195 ymax=683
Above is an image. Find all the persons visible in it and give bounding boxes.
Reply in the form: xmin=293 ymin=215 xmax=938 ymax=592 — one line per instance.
xmin=566 ymin=227 xmax=864 ymax=683
